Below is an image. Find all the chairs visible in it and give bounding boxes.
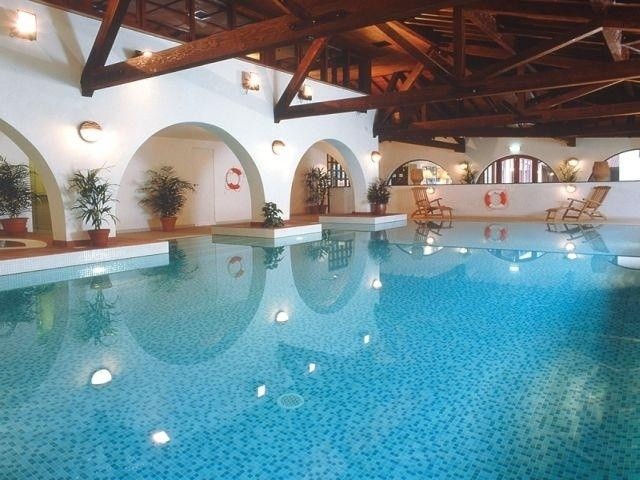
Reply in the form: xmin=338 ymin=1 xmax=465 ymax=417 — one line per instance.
xmin=547 ymin=220 xmax=610 ymax=250
xmin=409 ymin=183 xmax=453 ymax=223
xmin=542 ymin=185 xmax=611 ymax=221
xmin=396 ymin=222 xmax=449 ymax=258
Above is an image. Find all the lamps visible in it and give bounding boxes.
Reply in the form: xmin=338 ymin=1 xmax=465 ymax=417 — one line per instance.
xmin=371 ymin=276 xmax=383 ymax=293
xmin=371 ymin=151 xmax=381 ymax=165
xmin=272 ymin=140 xmax=286 ymax=157
xmin=459 ymin=160 xmax=467 ymax=170
xmin=92 ymin=369 xmax=111 ymax=390
xmin=78 ymin=120 xmax=102 ymax=143
xmin=567 ymin=156 xmax=581 ymax=167
xmin=274 ymin=309 xmax=290 ymax=325
xmin=133 ymin=49 xmax=315 ymax=104
xmin=7 ymin=8 xmax=39 ymax=43
xmin=563 ymin=240 xmax=576 ymax=251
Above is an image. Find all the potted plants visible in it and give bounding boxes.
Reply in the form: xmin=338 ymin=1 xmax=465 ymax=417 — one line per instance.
xmin=0 ymin=158 xmax=46 ymax=234
xmin=72 ymin=274 xmax=121 ymax=350
xmin=369 ymin=179 xmax=392 ymax=215
xmin=134 ymin=166 xmax=200 ymax=230
xmin=306 ymin=168 xmax=331 ymax=214
xmin=262 ymin=200 xmax=286 ymax=229
xmin=67 ymin=164 xmax=122 ymax=248
xmin=367 ymin=229 xmax=392 ymax=266
xmin=142 ymin=235 xmax=197 ymax=293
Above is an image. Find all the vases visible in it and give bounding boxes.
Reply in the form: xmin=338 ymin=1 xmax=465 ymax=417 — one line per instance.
xmin=592 ymin=161 xmax=612 ymax=182
xmin=409 ymin=168 xmax=425 ymax=186
